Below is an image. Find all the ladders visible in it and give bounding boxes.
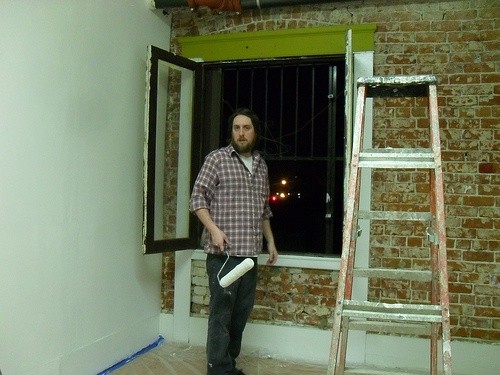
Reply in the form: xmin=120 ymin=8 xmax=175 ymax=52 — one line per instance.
xmin=326 ymin=76 xmax=453 ymax=374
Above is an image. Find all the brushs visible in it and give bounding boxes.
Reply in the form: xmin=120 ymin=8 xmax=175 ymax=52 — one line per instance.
xmin=217 ymin=242 xmax=254 ymax=288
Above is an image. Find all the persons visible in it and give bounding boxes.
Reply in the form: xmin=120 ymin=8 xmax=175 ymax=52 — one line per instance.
xmin=188 ymin=107 xmax=279 ymax=375
